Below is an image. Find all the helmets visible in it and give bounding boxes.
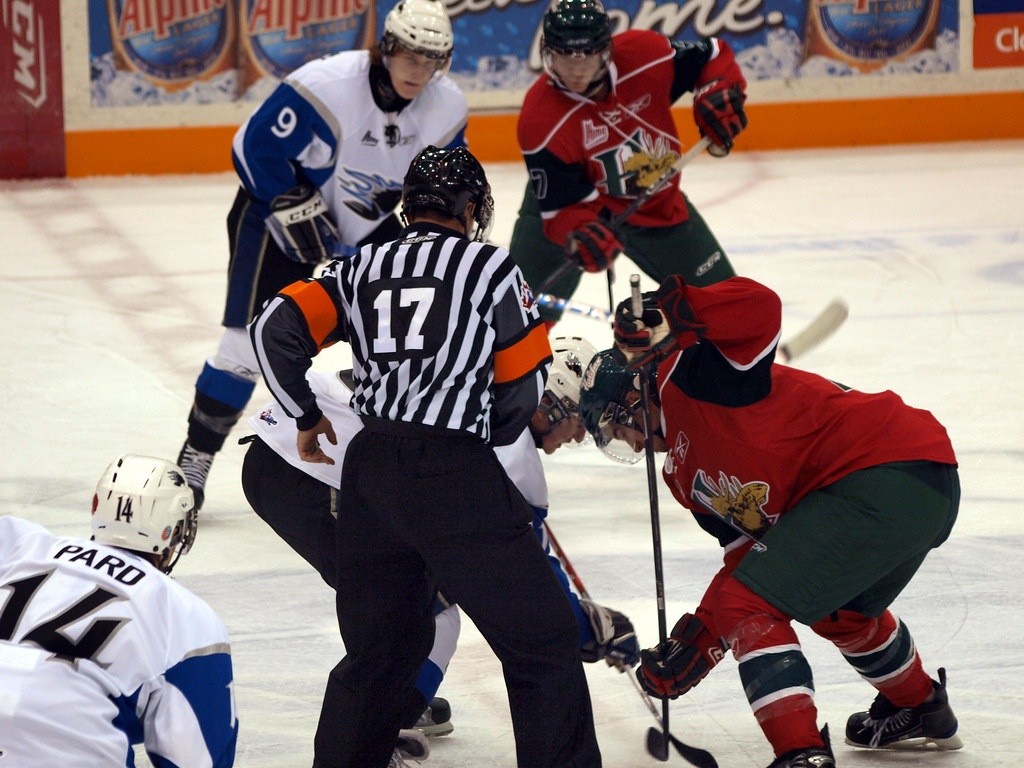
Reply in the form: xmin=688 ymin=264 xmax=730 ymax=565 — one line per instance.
xmin=402 ymin=144 xmax=491 ymax=235
xmin=543 ymin=0 xmax=611 ymax=88
xmin=382 ymin=0 xmax=453 ymax=85
xmin=92 ymin=455 xmax=197 ymax=555
xmin=578 ymin=345 xmax=662 ymax=464
xmin=544 ymin=335 xmax=597 ymax=420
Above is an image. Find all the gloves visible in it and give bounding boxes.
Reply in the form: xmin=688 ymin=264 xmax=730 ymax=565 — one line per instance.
xmin=265 ymin=184 xmax=339 ymax=263
xmin=615 ymin=291 xmax=673 ymax=350
xmin=580 ymin=598 xmax=641 ymax=673
xmin=636 ymin=614 xmax=723 ymax=699
xmin=568 ymin=219 xmax=624 ymax=272
xmin=693 ymin=78 xmax=747 ymax=156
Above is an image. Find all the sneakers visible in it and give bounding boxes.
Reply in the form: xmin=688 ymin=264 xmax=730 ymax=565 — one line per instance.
xmin=765 ymin=724 xmax=836 ymax=768
xmin=410 ymin=696 xmax=454 ymax=737
xmin=844 ymin=668 xmax=964 ymax=752
xmin=177 ymin=440 xmax=214 ymax=512
xmin=389 ymin=730 xmax=429 ymax=766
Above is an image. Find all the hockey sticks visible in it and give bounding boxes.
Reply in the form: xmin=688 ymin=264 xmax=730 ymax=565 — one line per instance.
xmin=630 ymin=274 xmax=670 ymax=762
xmin=544 ymin=520 xmax=719 ymax=768
xmin=533 ymin=290 xmax=849 ymax=364
xmin=538 ymin=135 xmax=713 ymax=293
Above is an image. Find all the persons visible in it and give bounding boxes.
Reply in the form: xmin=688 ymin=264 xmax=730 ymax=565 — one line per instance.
xmin=507 ymin=0 xmax=747 ymax=337
xmin=243 ymin=149 xmax=637 ymax=768
xmin=0 ymin=453 xmax=239 ymax=768
xmin=577 ymin=277 xmax=959 ymax=768
xmin=176 ymin=0 xmax=469 ymax=509
